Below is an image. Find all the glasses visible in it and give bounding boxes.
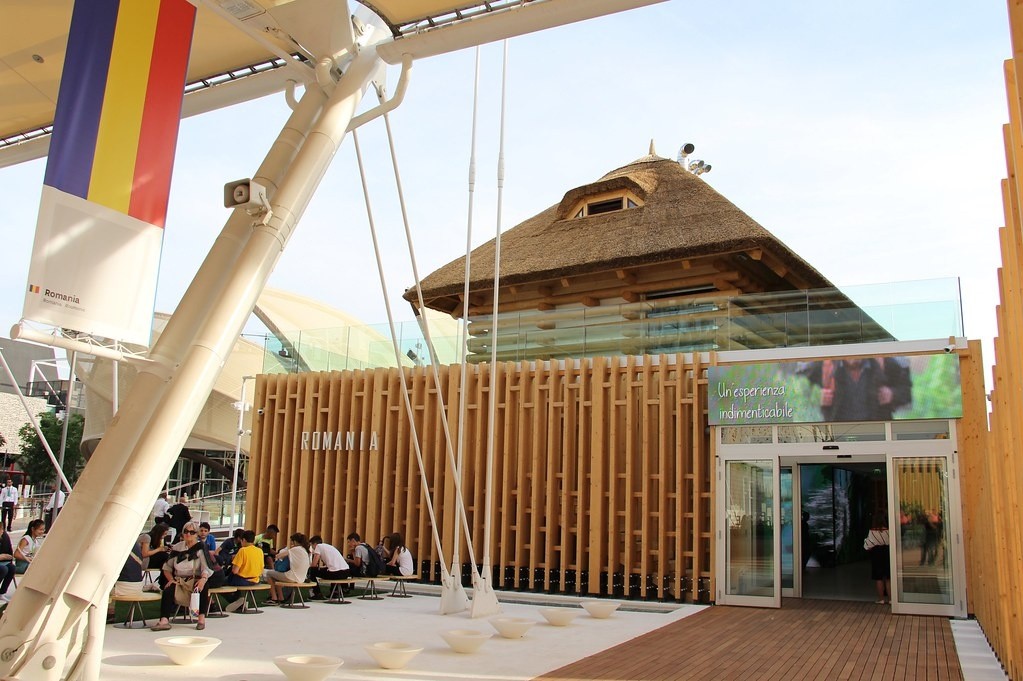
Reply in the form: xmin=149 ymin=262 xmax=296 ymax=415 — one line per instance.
xmin=184 ymin=528 xmax=197 ymax=535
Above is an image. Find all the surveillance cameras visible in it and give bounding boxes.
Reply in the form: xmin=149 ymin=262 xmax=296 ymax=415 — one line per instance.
xmin=258 ymin=409 xmax=264 ymax=414
xmin=943 ymin=344 xmax=955 ymax=353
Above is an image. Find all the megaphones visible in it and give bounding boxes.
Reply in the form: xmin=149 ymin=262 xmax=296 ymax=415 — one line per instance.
xmin=224 ymin=177 xmax=267 ymax=209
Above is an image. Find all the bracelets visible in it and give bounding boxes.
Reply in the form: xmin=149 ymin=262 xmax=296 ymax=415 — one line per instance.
xmin=202 ymin=577 xmax=208 ymax=582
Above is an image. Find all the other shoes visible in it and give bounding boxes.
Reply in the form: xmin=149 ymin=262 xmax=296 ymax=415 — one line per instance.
xmin=152 ymin=622 xmax=171 ymax=631
xmin=225 ymin=597 xmax=245 ymax=612
xmin=875 ymin=600 xmax=885 ymax=604
xmin=196 ymin=622 xmax=205 ymax=630
xmin=261 ymin=599 xmax=279 ymax=605
xmin=307 ymin=594 xmax=328 ymax=601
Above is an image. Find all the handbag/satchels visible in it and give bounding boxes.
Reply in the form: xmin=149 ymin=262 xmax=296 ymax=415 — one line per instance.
xmin=190 ymin=587 xmax=199 ymax=620
xmin=174 ymin=577 xmax=194 ymax=605
xmin=142 ymin=571 xmax=161 ymax=592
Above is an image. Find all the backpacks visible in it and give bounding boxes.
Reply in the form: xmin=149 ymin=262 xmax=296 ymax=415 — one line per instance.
xmin=356 ymin=542 xmax=385 ymax=578
xmin=274 ymin=556 xmax=289 ymax=573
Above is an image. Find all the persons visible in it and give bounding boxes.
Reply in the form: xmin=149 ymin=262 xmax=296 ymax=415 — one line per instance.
xmin=901 ymin=507 xmax=943 ymax=567
xmin=818 ymin=354 xmax=912 ymax=421
xmin=118 ymin=523 xmax=172 ymax=582
xmin=0 ymin=478 xmax=20 ymax=532
xmin=43 ymin=483 xmax=66 ymax=528
xmin=802 ymin=512 xmax=810 ymax=573
xmin=150 ymin=521 xmax=225 ymax=631
xmin=0 ymin=522 xmax=16 ymax=602
xmin=14 ymin=519 xmax=45 ymax=574
xmin=756 ymin=513 xmax=773 ymax=560
xmin=306 ymin=536 xmax=351 ymax=600
xmin=260 ymin=533 xmax=312 ymax=605
xmin=863 ymin=513 xmax=891 ymax=604
xmin=154 ymin=490 xmax=280 ymax=613
xmin=338 ymin=533 xmax=414 ymax=597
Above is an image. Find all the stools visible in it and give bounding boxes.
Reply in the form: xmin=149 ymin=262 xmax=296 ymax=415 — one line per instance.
xmin=8 ymin=567 xmax=418 ymax=628
